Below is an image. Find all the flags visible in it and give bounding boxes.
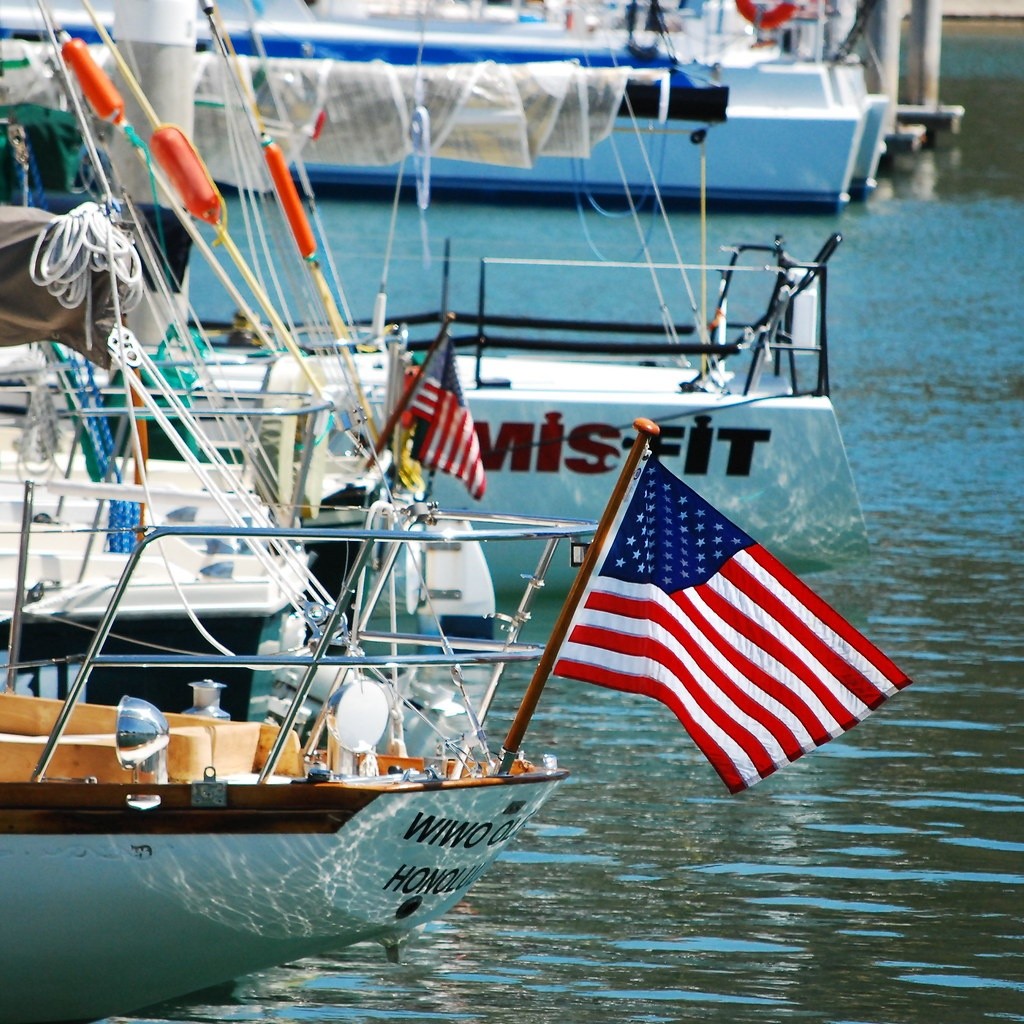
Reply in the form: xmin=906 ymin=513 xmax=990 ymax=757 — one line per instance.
xmin=387 ymin=337 xmax=488 ymax=502
xmin=553 ymin=453 xmax=915 ymax=795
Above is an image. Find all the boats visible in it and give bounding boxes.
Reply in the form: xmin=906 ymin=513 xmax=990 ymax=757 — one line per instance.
xmin=0 ymin=0 xmax=900 ymax=1024
